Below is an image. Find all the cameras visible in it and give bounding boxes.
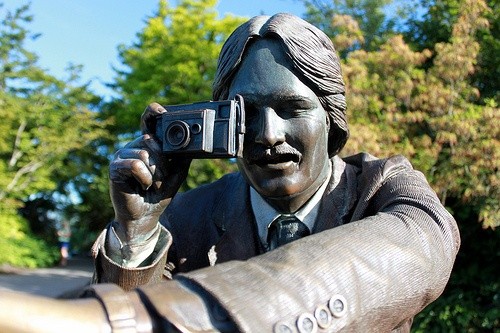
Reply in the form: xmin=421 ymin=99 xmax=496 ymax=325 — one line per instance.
xmin=151 ymin=101 xmax=244 ymax=159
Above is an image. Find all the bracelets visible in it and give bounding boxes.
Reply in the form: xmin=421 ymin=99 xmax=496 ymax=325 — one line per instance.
xmin=80 ymin=283 xmax=139 ymax=333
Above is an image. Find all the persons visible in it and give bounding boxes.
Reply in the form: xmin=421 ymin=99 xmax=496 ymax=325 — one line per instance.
xmin=0 ymin=11 xmax=462 ymax=333
xmin=56 ymin=222 xmax=71 ymax=267
